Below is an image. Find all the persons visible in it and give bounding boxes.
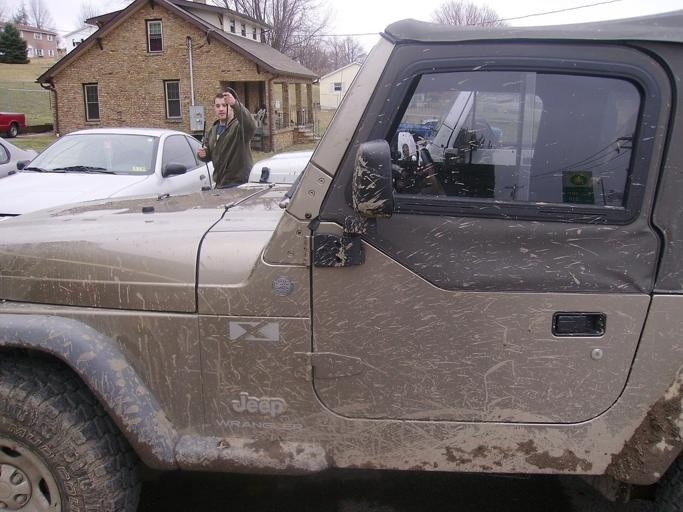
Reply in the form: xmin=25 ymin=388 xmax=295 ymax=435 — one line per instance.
xmin=197 ymin=93 xmax=257 ymax=188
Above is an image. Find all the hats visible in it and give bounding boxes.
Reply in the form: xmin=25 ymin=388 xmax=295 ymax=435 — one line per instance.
xmin=0 ymin=135 xmax=39 ymax=180
xmin=0 ymin=128 xmax=215 ymax=225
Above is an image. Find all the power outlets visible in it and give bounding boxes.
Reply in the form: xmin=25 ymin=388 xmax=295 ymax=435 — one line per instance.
xmin=0 ymin=111 xmax=27 ymax=138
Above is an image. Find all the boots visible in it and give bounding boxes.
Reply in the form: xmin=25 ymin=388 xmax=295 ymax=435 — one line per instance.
xmin=248 ymin=88 xmax=541 ymax=188
xmin=0 ymin=17 xmax=681 ymax=512
